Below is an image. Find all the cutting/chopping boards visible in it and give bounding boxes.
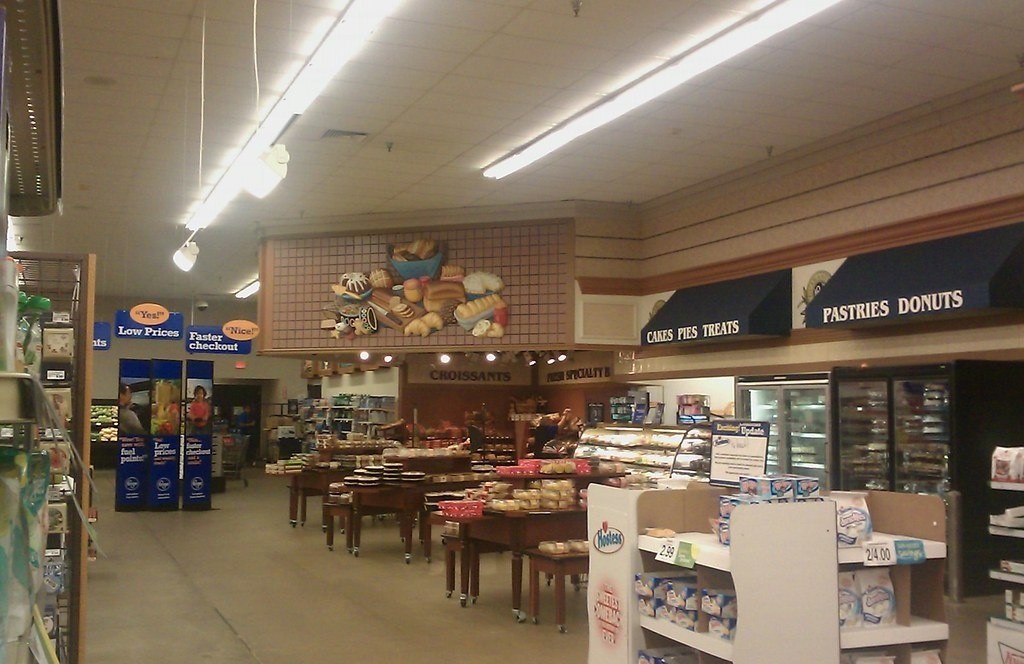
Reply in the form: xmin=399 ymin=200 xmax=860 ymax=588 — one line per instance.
xmin=353 ymin=290 xmax=426 ymax=331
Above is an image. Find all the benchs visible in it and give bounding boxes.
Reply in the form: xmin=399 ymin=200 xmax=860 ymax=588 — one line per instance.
xmin=325 ymin=503 xmax=353 ymax=554
xmin=286 ymin=485 xmax=328 ymax=527
xmin=524 ymin=545 xmax=588 ymax=634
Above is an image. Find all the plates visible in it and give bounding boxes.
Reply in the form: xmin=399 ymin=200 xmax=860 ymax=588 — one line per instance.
xmin=343 ymin=463 xmax=426 ymax=487
xmin=336 ymin=288 xmax=373 ymax=302
xmin=339 ymin=304 xmax=362 ymax=318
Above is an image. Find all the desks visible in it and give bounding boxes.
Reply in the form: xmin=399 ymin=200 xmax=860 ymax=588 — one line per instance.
xmin=285 ymin=445 xmax=403 ymax=532
xmin=429 ymin=469 xmax=632 ymax=624
xmin=343 ymin=455 xmax=526 ymax=563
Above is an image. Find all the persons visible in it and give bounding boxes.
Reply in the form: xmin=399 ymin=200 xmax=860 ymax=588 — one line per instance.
xmin=187 ymin=386 xmax=211 ymax=436
xmin=235 ymin=403 xmax=256 ymax=441
xmin=119 ymin=385 xmax=148 ymax=437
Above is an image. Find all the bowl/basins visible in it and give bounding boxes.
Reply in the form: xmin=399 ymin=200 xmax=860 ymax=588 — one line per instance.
xmin=424 ymin=493 xmax=466 ymax=511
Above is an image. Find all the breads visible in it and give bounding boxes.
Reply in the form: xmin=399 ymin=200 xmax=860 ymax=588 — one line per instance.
xmin=332 ymin=238 xmax=508 ymax=337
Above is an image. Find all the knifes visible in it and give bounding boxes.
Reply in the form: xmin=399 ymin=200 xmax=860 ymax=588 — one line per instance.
xmin=367 ymin=300 xmax=404 ymax=325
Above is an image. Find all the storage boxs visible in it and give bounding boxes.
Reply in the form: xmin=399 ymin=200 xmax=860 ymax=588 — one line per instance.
xmin=668 ymin=605 xmax=708 ymax=632
xmin=708 ymin=615 xmax=736 ymax=644
xmin=772 ymin=474 xmax=820 ymax=498
xmin=717 ymin=518 xmax=732 ymax=546
xmin=666 ymin=579 xmax=699 ymax=611
xmin=719 ymin=494 xmax=761 ymax=522
xmin=701 ymin=587 xmax=738 ymax=618
xmin=638 ymin=646 xmax=697 ymax=664
xmin=738 ymin=475 xmax=794 ymax=498
xmin=443 ymin=509 xmax=482 ymax=519
xmin=638 ymin=594 xmax=667 ymax=619
xmin=438 ymin=500 xmax=483 ymax=511
xmin=635 ymin=570 xmax=702 ymax=599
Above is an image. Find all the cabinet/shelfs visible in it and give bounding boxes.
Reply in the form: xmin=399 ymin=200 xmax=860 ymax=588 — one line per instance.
xmin=587 ymin=472 xmax=949 ymax=664
xmin=407 ymin=424 xmax=516 ymax=466
xmin=299 ymin=393 xmax=396 ymax=454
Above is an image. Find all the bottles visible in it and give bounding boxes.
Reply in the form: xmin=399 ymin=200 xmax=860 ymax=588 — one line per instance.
xmin=336 ymin=394 xmax=367 ymax=409
xmin=408 ymin=437 xmax=467 ymax=448
